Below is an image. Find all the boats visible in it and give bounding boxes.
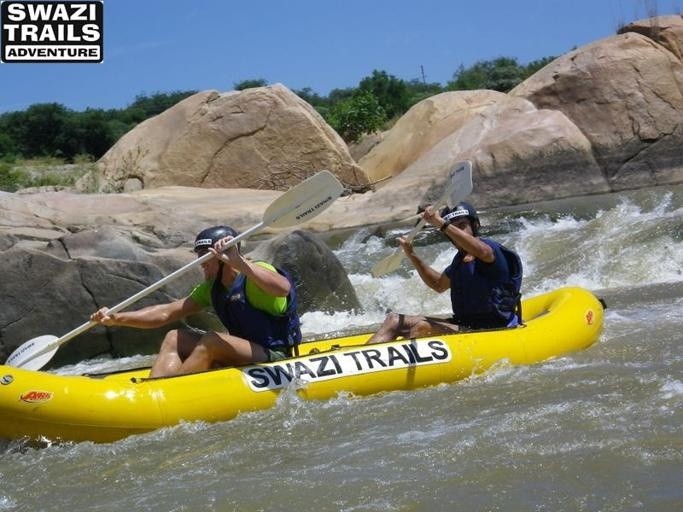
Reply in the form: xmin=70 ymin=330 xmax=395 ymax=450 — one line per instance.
xmin=0 ymin=287 xmax=605 ymax=449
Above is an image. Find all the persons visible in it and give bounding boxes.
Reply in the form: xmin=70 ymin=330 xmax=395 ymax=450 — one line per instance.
xmin=309 ymin=201 xmax=525 ymax=355
xmin=90 ymin=225 xmax=303 ymax=379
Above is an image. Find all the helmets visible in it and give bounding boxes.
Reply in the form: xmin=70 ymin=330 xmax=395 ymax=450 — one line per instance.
xmin=441 ymin=201 xmax=481 ymax=231
xmin=195 ymin=226 xmax=241 ymax=258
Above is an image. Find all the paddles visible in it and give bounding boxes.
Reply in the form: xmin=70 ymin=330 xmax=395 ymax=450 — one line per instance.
xmin=4 ymin=171 xmax=344 ymax=370
xmin=371 ymin=160 xmax=474 ymax=277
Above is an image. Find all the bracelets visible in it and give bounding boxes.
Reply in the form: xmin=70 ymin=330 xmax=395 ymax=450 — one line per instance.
xmin=441 ymin=220 xmax=451 ymax=233
